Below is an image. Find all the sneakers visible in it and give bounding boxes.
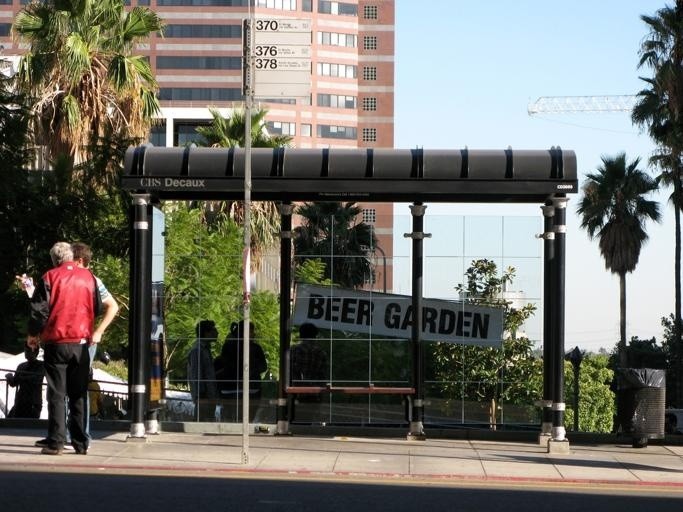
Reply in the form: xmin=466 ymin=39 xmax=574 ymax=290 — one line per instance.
xmin=37 ymin=439 xmax=89 ymax=455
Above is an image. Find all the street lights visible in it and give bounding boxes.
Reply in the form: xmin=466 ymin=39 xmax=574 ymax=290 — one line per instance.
xmin=569 ymin=346 xmax=583 ymax=431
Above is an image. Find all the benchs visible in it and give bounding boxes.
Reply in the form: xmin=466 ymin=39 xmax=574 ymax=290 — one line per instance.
xmin=194 ymin=396 xmax=297 ymax=411
xmin=285 ymin=384 xmax=416 ymax=397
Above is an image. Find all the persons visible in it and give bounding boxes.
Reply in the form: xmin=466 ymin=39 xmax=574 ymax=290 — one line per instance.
xmin=0 ymin=241 xmax=120 ymax=456
xmin=186 ymin=319 xmax=219 ymax=423
xmin=219 ymin=319 xmax=267 ymax=401
xmin=289 ymin=322 xmax=330 ymax=404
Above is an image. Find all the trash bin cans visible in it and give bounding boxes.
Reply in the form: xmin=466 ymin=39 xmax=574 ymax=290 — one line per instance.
xmin=617 ymin=368 xmax=666 ymax=440
